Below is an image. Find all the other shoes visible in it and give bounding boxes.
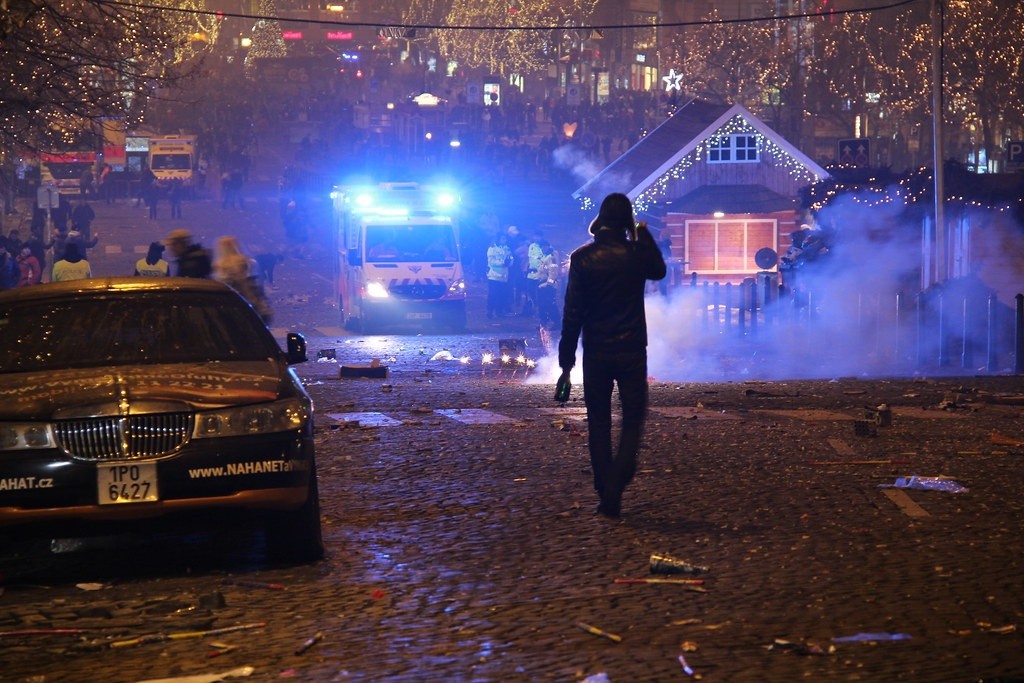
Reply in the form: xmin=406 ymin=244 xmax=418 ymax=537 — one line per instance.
xmin=596 ymin=489 xmax=621 ymax=515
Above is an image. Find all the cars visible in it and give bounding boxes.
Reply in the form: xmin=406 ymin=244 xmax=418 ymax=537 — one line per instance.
xmin=0 ymin=275 xmax=327 ymax=573
xmin=52 ymin=161 xmax=113 ymax=198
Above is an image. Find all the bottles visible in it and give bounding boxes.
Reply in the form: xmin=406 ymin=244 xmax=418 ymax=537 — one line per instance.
xmin=649 ymin=551 xmax=711 ymax=575
xmin=554 ymin=366 xmax=573 ymax=401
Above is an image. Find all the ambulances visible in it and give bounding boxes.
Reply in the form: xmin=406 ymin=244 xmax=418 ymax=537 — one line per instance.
xmin=330 ymin=184 xmax=468 ymax=330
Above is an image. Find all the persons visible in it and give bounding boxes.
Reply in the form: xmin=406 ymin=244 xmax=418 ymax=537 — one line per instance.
xmin=104 ymin=168 xmax=117 ymax=205
xmin=78 ymin=167 xmax=96 ymax=200
xmin=275 ymin=120 xmax=405 ymax=259
xmin=555 ymin=192 xmax=666 ymax=518
xmin=194 ymin=128 xmax=250 ymax=211
xmin=48 ymin=238 xmax=92 ymax=282
xmin=135 ymin=167 xmax=185 ymax=220
xmin=423 ymin=83 xmax=652 ymax=186
xmin=160 ymin=228 xmax=213 ymax=280
xmin=212 ymin=237 xmax=271 ymax=317
xmin=484 ymin=224 xmax=562 ymax=334
xmin=131 ymin=242 xmax=171 ymax=277
xmin=0 ymin=190 xmax=95 ymax=291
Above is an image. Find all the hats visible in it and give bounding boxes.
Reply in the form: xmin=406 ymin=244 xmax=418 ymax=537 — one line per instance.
xmin=159 ymin=229 xmax=191 ymax=248
xmin=587 ymin=193 xmax=636 ymax=242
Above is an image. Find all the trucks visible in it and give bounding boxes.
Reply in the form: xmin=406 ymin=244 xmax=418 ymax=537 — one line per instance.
xmin=147 ymin=133 xmax=200 ymax=199
